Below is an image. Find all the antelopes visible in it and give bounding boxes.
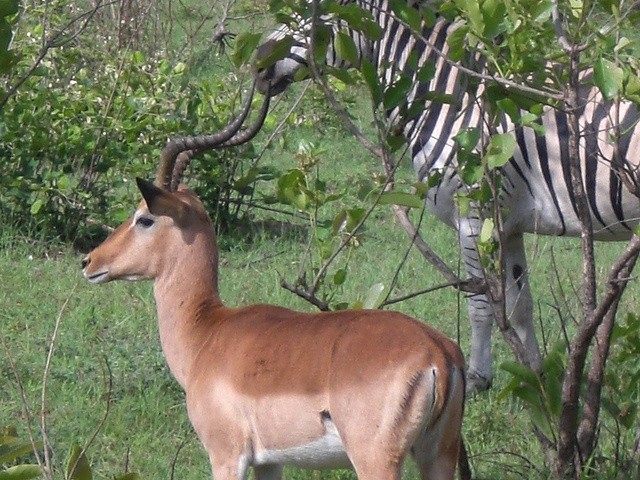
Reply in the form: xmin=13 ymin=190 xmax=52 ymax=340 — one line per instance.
xmin=81 ymin=78 xmax=473 ymax=479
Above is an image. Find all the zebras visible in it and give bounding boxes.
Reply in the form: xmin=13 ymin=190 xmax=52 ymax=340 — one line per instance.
xmin=252 ymin=0 xmax=639 ymax=396
